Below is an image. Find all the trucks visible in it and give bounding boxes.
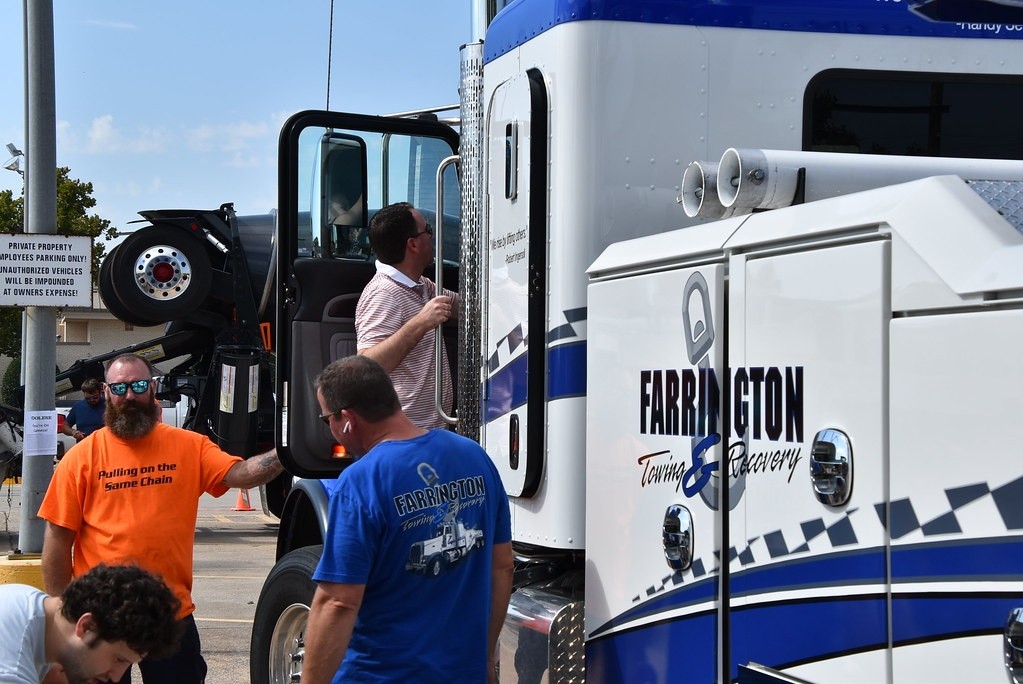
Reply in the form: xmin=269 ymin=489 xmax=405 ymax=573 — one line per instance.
xmin=249 ymin=0 xmax=1023 ymax=684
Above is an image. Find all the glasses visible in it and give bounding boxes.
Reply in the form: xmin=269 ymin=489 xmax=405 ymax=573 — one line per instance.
xmin=319 ymin=409 xmax=349 ymax=426
xmin=411 ymin=223 xmax=433 ymax=239
xmin=102 ymin=379 xmax=153 ymax=395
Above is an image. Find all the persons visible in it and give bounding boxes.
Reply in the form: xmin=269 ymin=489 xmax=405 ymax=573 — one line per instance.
xmin=299 ymin=355 xmax=513 ymax=684
xmin=62 ymin=378 xmax=106 ymax=443
xmin=354 ymin=202 xmax=459 ymax=430
xmin=37 ymin=352 xmax=284 ymax=684
xmin=0 ymin=564 xmax=175 ymax=684
